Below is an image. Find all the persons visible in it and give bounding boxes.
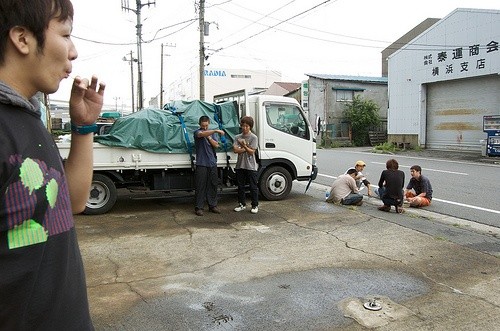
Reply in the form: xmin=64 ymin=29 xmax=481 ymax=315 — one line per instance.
xmin=232 ymin=115 xmax=259 ymax=213
xmin=403 ymin=164 xmax=433 ymax=207
xmin=344 ymin=161 xmax=373 ymax=196
xmin=193 ymin=115 xmax=225 ymax=216
xmin=329 ymin=169 xmax=365 ymax=207
xmin=1 ymin=0 xmax=107 ymax=331
xmin=376 ymin=159 xmax=406 ymax=213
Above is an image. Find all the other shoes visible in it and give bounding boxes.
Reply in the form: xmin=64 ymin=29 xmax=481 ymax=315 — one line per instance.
xmin=357 ymin=202 xmax=363 ymax=205
xmin=378 ymin=205 xmax=390 ymax=211
xmin=196 ymin=209 xmax=203 ymax=215
xmin=396 ymin=207 xmax=405 ymax=213
xmin=209 ymin=206 xmax=221 ymax=213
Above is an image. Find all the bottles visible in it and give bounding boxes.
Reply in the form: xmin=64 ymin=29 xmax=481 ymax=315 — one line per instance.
xmin=325 ymin=191 xmax=329 ymax=199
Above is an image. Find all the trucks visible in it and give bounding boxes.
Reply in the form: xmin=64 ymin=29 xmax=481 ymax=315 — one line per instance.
xmin=53 ymin=88 xmax=319 ymax=215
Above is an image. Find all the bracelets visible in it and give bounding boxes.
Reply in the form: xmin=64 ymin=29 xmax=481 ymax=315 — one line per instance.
xmin=70 ymin=122 xmax=98 ymax=135
xmin=208 ymin=137 xmax=213 ymax=141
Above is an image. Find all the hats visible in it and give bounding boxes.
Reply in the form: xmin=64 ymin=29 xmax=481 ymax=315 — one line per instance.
xmin=356 ymin=161 xmax=366 ymax=166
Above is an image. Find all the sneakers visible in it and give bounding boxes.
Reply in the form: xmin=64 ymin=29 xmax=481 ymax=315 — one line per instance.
xmin=251 ymin=206 xmax=258 ymax=213
xmin=234 ymin=203 xmax=246 ymax=212
